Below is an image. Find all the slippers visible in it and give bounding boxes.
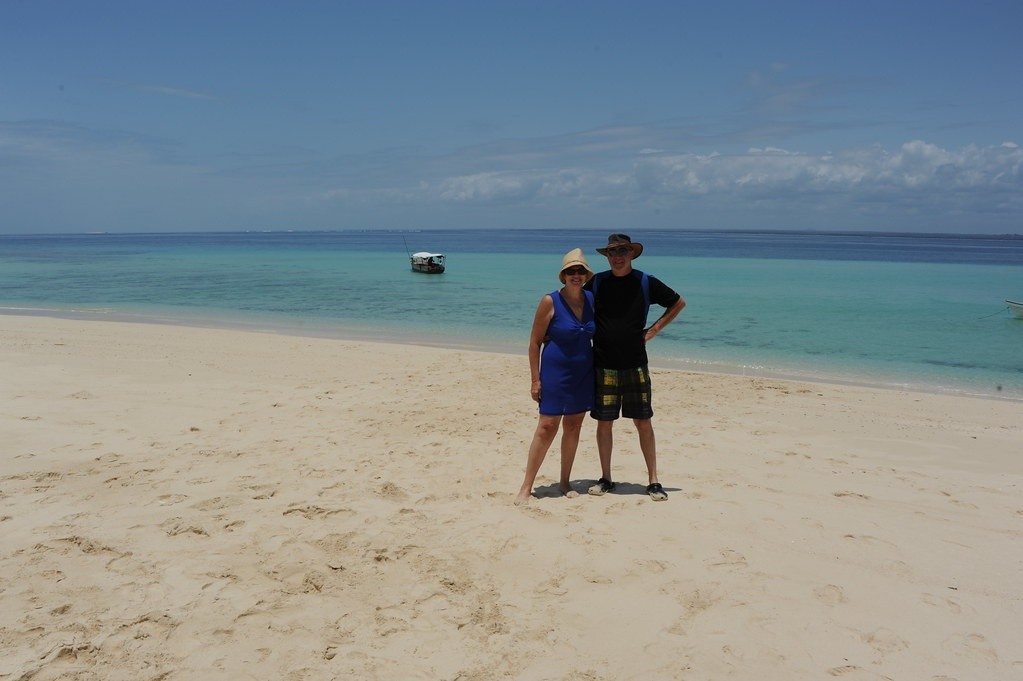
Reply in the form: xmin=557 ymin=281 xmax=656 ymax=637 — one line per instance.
xmin=589 ymin=478 xmax=616 ymax=495
xmin=646 ymin=482 xmax=668 ymax=501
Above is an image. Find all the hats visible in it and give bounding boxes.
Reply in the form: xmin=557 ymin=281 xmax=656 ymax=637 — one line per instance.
xmin=596 ymin=234 xmax=643 ymax=260
xmin=558 ymin=248 xmax=593 ymax=284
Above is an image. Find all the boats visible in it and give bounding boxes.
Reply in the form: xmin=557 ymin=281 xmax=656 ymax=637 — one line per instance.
xmin=410 ymin=251 xmax=446 ymax=274
xmin=1003 ymin=299 xmax=1023 ymax=319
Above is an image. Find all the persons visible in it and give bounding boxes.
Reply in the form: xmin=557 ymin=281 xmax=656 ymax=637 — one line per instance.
xmin=514 ymin=249 xmax=595 ymax=506
xmin=428 ymin=257 xmax=435 ymax=266
xmin=581 ymin=233 xmax=685 ymax=501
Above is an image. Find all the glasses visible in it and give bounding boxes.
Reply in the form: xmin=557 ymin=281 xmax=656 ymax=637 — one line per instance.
xmin=565 ymin=267 xmax=587 ymax=275
xmin=607 ymin=247 xmax=632 ymax=257
